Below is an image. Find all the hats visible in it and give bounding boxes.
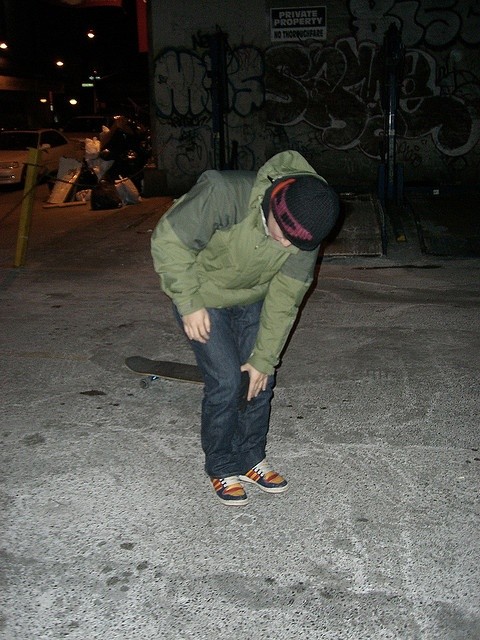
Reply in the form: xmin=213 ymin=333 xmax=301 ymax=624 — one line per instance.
xmin=270 ymin=174 xmax=338 ymax=251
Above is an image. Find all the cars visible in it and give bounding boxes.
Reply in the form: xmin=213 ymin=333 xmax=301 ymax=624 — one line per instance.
xmin=58 ymin=115 xmax=110 ymax=153
xmin=0 ymin=127 xmax=80 ymax=186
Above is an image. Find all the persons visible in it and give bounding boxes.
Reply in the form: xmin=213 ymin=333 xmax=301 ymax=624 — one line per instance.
xmin=150 ymin=149 xmax=341 ymax=508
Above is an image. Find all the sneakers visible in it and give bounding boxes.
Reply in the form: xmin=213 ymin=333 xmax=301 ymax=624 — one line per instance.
xmin=239 ymin=458 xmax=290 ymax=494
xmin=210 ymin=476 xmax=249 ymax=506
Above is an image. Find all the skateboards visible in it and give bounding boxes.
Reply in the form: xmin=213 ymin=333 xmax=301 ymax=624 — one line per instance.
xmin=125 ymin=355 xmax=205 ymax=389
